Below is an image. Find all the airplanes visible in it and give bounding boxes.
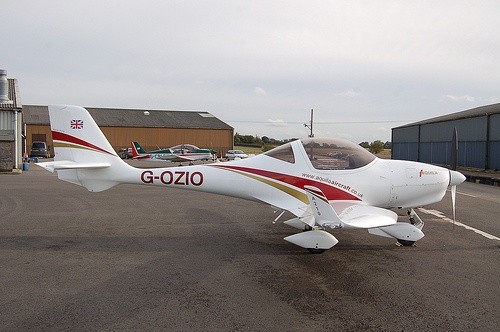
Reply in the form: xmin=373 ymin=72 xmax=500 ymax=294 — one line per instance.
xmin=131 ymin=141 xmax=216 ymax=166
xmin=33 ymin=104 xmax=466 ymax=250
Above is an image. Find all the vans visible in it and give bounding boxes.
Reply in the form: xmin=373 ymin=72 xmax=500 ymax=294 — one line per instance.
xmin=30 ymin=140 xmax=50 ymax=158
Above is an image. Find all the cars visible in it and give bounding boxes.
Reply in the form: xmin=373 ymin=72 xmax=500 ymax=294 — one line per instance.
xmin=225 ymin=149 xmax=248 ymax=161
xmin=126 ymin=147 xmax=133 ymax=158
xmin=117 ymin=146 xmax=128 ymax=158
